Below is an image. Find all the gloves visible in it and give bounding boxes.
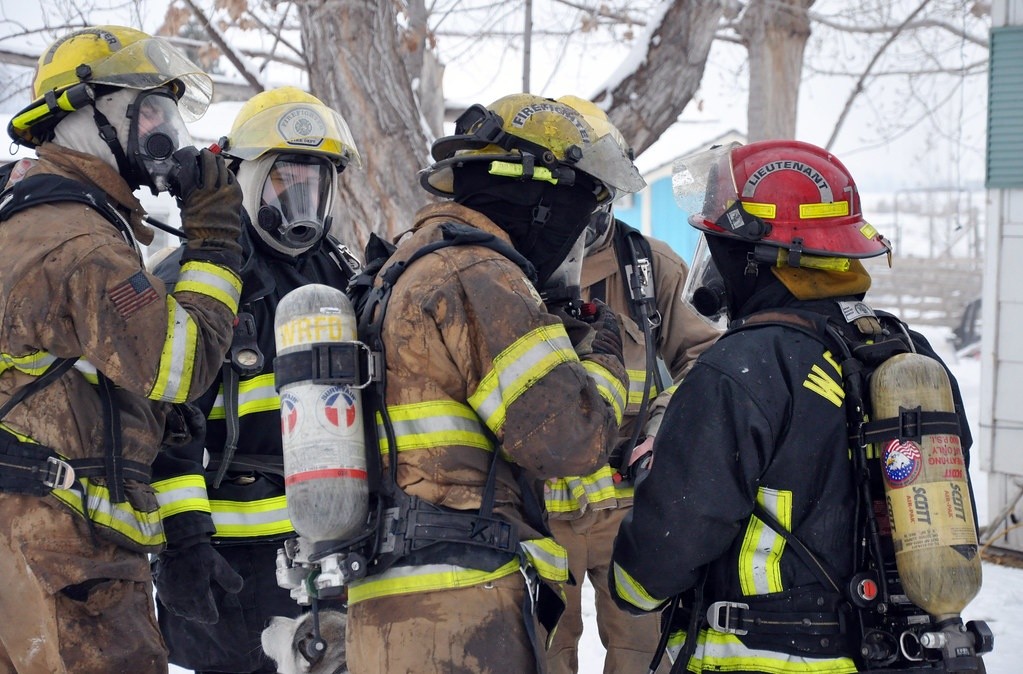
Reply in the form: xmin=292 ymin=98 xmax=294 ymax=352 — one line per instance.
xmin=550 ymin=297 xmax=623 ymax=362
xmin=149 ymin=543 xmax=243 ymax=623
xmin=173 ymin=148 xmax=245 ymax=273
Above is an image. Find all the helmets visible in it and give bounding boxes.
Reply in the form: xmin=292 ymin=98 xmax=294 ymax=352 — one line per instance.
xmin=553 ymin=95 xmax=635 ymax=162
xmin=6 ymin=24 xmax=185 ymax=154
xmin=218 ymin=86 xmax=349 ymax=174
xmin=419 ymin=93 xmax=618 ymax=214
xmin=687 ymin=140 xmax=891 ymax=271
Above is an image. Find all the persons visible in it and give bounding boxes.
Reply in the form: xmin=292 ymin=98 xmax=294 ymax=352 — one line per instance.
xmin=446 ymin=98 xmax=722 ymax=674
xmin=127 ymin=86 xmax=369 ymax=674
xmin=606 ymin=138 xmax=977 ymax=674
xmin=336 ymin=93 xmax=629 ymax=674
xmin=0 ymin=28 xmax=250 ymax=674
